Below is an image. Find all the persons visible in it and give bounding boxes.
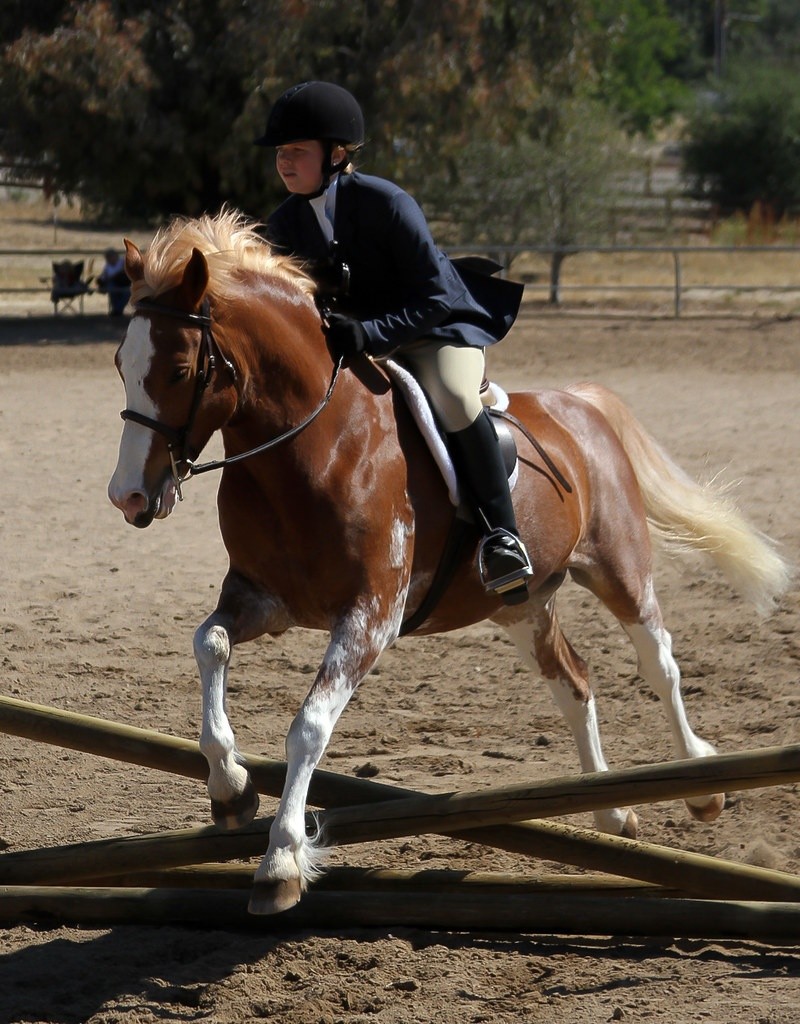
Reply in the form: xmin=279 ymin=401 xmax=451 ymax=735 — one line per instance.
xmin=257 ymin=84 xmax=530 ymax=605
xmin=96 ymin=249 xmax=131 ymax=317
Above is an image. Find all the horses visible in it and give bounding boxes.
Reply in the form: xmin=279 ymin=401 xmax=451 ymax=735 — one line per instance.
xmin=108 ymin=201 xmax=783 ymax=916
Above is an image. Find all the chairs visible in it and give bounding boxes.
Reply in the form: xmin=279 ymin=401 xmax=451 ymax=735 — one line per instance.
xmin=50 ymin=260 xmax=93 ymax=315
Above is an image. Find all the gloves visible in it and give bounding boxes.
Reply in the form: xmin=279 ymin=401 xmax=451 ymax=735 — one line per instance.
xmin=322 ymin=312 xmax=365 ymax=361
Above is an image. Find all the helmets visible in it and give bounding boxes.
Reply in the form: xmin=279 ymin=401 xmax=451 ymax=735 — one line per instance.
xmin=265 ymin=81 xmax=365 ymax=145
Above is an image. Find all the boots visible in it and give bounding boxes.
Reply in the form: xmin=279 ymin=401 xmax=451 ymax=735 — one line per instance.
xmin=441 ymin=407 xmax=528 ymax=605
